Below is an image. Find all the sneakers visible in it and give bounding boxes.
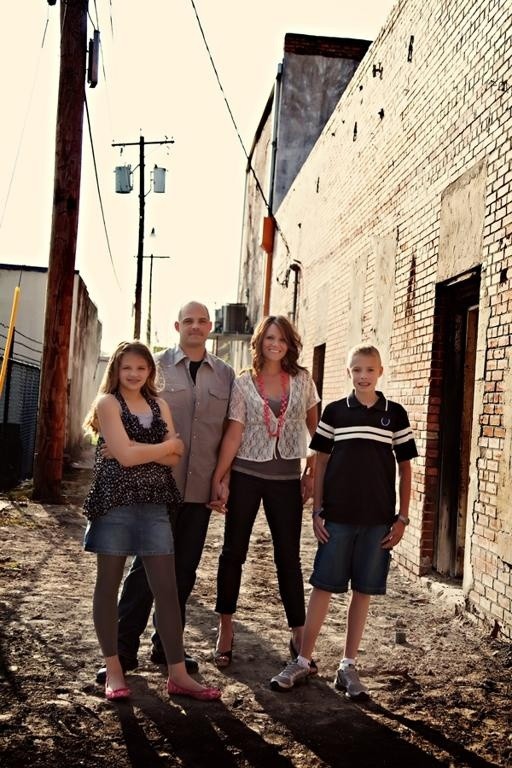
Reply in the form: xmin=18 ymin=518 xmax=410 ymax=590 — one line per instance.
xmin=269 ymin=658 xmax=312 ymax=692
xmin=334 ymin=667 xmax=368 ymax=702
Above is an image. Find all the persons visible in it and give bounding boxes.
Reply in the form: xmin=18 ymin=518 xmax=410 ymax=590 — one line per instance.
xmin=209 ymin=314 xmax=320 ymax=674
xmin=97 ymin=302 xmax=236 ymax=686
xmin=89 ymin=339 xmax=222 ymax=700
xmin=271 ymin=346 xmax=419 ymax=698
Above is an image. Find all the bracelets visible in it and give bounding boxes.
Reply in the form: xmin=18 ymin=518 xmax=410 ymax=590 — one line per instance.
xmin=312 ymin=509 xmax=322 ymax=519
xmin=304 ymin=467 xmax=314 ymax=477
xmin=395 ymin=514 xmax=411 ymax=526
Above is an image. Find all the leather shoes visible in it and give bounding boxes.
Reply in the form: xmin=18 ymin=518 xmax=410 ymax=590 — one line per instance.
xmin=103 ymin=672 xmax=131 ymax=700
xmin=147 ymin=651 xmax=200 ymax=674
xmin=165 ymin=676 xmax=221 ymax=701
xmin=97 ymin=655 xmax=139 ymax=683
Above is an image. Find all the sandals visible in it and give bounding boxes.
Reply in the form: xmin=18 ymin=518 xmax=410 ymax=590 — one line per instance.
xmin=212 ymin=629 xmax=235 ymax=668
xmin=287 ymin=638 xmax=319 ymax=674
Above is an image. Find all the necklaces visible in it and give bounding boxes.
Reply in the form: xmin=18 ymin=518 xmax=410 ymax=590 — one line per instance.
xmin=258 ymin=369 xmax=289 ymax=437
